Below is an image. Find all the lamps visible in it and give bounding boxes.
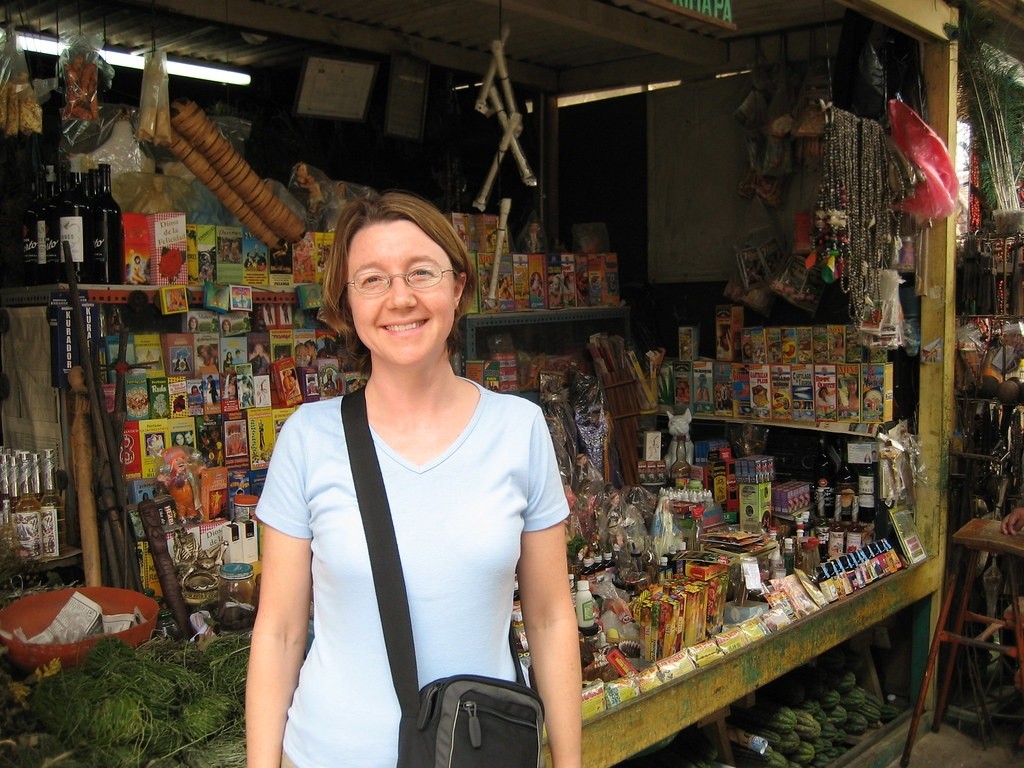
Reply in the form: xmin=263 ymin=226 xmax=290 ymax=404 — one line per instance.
xmin=0 ymin=26 xmax=252 ymax=86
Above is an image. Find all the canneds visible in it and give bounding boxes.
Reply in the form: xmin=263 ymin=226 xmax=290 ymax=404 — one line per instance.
xmin=218 ymin=562 xmax=258 ymax=630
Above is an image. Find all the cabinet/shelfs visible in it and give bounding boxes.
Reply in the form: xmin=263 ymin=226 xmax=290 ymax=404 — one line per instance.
xmin=74 ymin=280 xmax=935 ymax=768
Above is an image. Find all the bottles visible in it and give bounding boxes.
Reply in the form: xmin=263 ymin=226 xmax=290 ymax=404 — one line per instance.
xmin=20 ymin=164 xmax=124 ymax=287
xmin=0 ymin=448 xmax=65 ymax=560
xmin=576 ymin=486 xmax=886 ymax=637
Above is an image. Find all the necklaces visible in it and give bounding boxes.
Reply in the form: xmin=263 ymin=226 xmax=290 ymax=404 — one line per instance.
xmin=811 ymin=106 xmax=905 ymax=323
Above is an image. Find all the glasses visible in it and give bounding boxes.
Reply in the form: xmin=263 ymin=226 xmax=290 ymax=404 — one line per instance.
xmin=345 ymin=265 xmax=458 ymax=295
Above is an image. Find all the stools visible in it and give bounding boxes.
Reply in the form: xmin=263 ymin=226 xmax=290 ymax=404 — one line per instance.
xmin=899 ymin=515 xmax=1024 ymax=768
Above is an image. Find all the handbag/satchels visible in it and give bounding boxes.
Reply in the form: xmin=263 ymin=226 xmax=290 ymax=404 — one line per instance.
xmin=576 ymin=393 xmax=608 ymax=475
xmin=397 ymin=674 xmax=545 ymax=768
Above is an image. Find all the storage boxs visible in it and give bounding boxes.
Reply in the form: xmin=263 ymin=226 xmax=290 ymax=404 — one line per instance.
xmin=103 ymin=210 xmax=894 ymax=715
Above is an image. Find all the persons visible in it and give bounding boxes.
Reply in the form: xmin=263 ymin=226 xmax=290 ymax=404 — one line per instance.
xmin=521 ymin=218 xmax=544 ymax=254
xmin=500 ymin=272 xmax=616 ymax=304
xmin=157 ymin=448 xmax=206 ymax=523
xmin=245 ymin=188 xmax=583 ymax=768
xmin=677 ymin=326 xmax=860 ymax=412
xmin=606 ymin=494 xmax=620 ymax=547
xmin=132 ymin=240 xmax=370 ymax=498
